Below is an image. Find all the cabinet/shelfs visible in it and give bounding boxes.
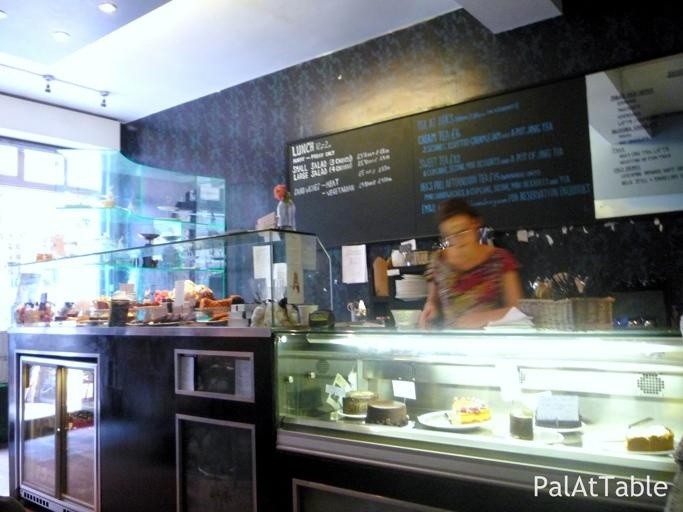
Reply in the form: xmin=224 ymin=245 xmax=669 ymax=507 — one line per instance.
xmin=1 ymin=228 xmax=682 ymax=512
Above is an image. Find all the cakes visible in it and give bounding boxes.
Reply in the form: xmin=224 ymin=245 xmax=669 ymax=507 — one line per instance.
xmin=364 ymin=400 xmax=408 ymax=427
xmin=625 ymin=424 xmax=672 ymax=450
xmin=343 ymin=391 xmax=379 ymax=414
xmin=509 ymin=407 xmax=534 ymax=441
xmin=453 ymin=398 xmax=492 ymax=424
xmin=535 ymin=395 xmax=581 ymax=428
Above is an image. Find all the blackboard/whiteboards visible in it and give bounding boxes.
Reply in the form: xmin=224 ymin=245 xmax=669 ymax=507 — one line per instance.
xmin=288 ymin=52 xmax=683 ymax=247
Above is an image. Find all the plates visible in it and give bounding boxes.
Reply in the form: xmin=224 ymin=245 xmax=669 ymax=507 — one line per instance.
xmin=533 ymin=421 xmax=585 ymax=444
xmin=338 ymin=409 xmax=368 ymax=420
xmin=393 ymin=273 xmax=428 ymax=302
xmin=615 ymin=441 xmax=678 ymax=456
xmin=417 ymin=409 xmax=484 ymax=430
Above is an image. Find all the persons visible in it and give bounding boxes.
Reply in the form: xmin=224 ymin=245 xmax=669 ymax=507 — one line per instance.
xmin=418 ymin=196 xmax=521 ymax=329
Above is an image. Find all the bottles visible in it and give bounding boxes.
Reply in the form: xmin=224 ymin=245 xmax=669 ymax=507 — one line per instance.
xmin=275 ymin=192 xmax=297 ymax=232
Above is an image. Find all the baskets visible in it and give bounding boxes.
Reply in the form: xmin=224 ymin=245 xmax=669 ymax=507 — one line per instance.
xmin=518 ymin=295 xmax=616 ymax=331
